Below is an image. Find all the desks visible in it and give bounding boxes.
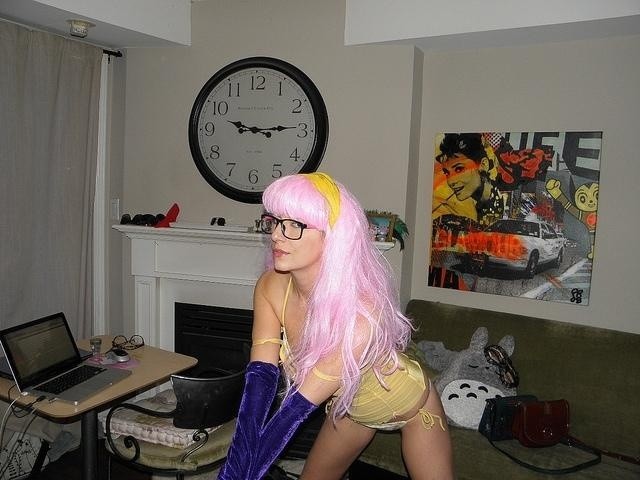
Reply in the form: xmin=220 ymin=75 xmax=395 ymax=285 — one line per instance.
xmin=2 ymin=334 xmax=198 ymax=478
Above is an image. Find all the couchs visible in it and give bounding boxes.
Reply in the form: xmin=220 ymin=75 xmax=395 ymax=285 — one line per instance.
xmin=357 ymin=300 xmax=639 ymax=480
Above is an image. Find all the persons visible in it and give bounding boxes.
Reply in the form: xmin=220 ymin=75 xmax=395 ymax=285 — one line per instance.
xmin=434 ymin=134 xmax=536 ymax=222
xmin=213 ymin=173 xmax=454 ymax=480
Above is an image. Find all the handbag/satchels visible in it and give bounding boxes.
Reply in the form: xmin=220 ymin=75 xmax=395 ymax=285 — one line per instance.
xmin=478 ymin=394 xmax=570 ymax=448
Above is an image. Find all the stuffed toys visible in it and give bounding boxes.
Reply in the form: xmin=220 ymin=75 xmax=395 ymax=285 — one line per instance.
xmin=416 ymin=327 xmax=518 ymax=430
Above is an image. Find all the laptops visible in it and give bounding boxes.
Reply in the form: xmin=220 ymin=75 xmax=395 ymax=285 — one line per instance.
xmin=0 ymin=311 xmax=132 ymax=407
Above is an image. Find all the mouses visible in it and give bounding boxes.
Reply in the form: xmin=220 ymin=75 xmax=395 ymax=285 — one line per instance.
xmin=107 ymin=348 xmax=129 ymax=362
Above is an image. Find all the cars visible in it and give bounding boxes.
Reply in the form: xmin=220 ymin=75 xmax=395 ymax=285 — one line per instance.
xmin=454 ymin=219 xmax=570 ymax=277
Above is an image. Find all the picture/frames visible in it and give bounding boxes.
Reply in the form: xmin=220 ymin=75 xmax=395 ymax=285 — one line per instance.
xmin=362 ymin=213 xmax=396 ymax=241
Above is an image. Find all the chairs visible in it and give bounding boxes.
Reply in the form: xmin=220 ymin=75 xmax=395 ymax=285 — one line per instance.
xmin=99 ymin=341 xmax=250 ymax=478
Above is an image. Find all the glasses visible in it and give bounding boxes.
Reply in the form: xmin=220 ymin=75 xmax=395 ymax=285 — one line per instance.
xmin=260 ymin=214 xmax=315 ymax=240
xmin=121 ymin=213 xmax=164 ymax=225
xmin=105 ymin=334 xmax=144 ymax=357
xmin=210 ymin=218 xmax=226 ymax=226
xmin=485 ymin=344 xmax=520 ymax=387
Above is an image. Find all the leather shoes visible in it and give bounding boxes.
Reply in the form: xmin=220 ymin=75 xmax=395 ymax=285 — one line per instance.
xmin=156 ymin=204 xmax=180 ymax=227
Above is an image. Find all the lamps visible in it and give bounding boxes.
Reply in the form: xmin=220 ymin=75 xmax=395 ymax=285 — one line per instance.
xmin=67 ymin=18 xmax=96 ymax=39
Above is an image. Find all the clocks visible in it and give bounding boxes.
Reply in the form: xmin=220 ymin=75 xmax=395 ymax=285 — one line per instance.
xmin=187 ymin=55 xmax=330 ymax=206
